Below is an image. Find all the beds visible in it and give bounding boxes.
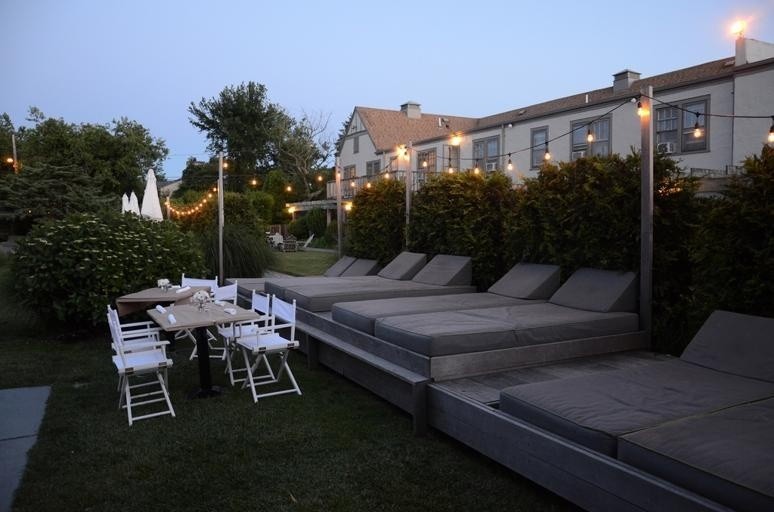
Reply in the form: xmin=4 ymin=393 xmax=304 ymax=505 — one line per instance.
xmin=328 ymin=263 xmax=642 ymax=437
xmin=499 ymin=310 xmax=773 ymax=510
xmin=228 ymin=254 xmax=476 ymax=348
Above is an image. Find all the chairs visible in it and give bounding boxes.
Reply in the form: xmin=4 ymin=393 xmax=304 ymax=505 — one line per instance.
xmin=106 ymin=273 xmax=302 ymax=426
